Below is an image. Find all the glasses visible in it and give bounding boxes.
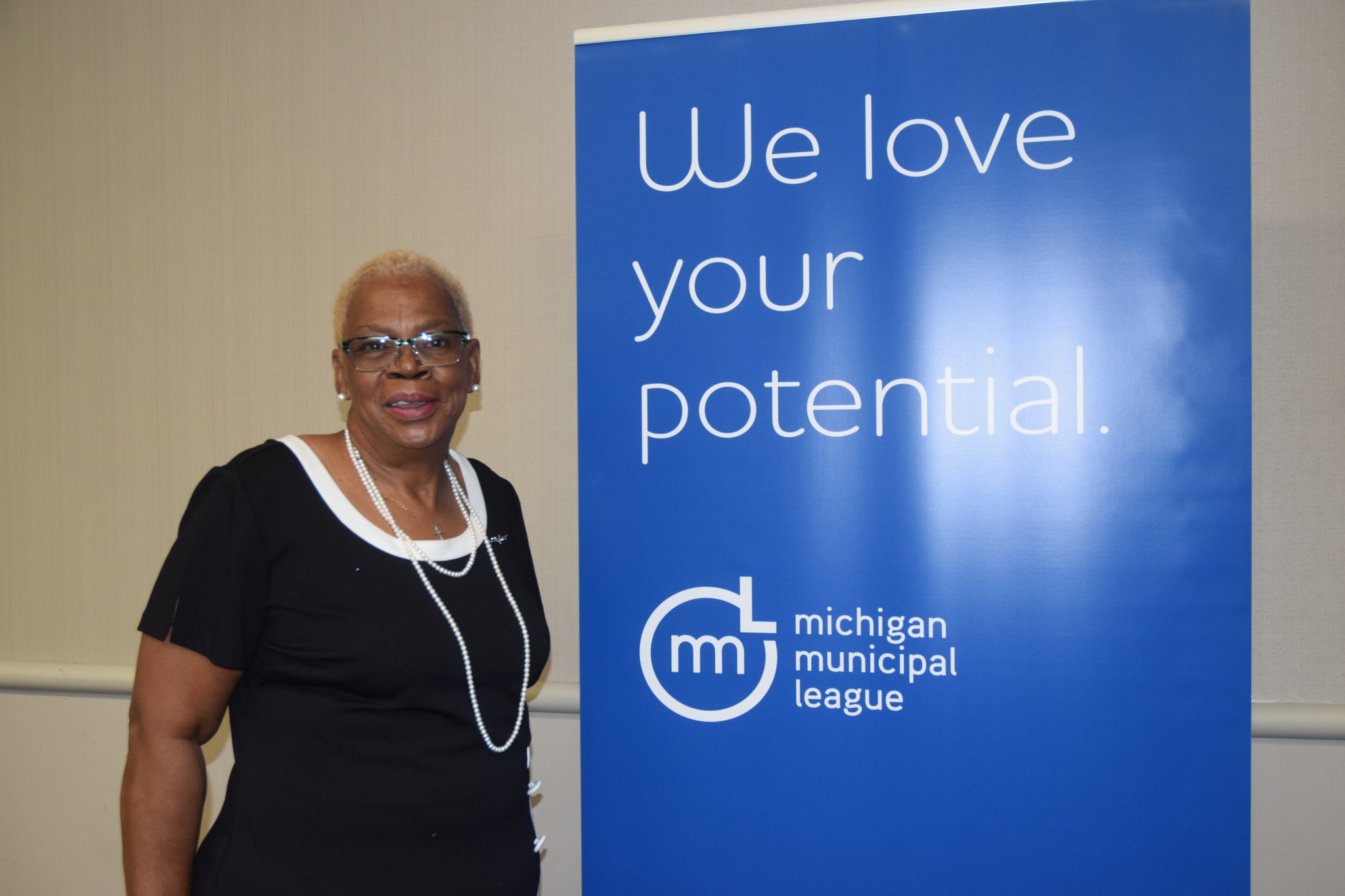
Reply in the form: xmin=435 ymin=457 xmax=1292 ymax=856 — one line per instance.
xmin=340 ymin=330 xmax=470 ymax=372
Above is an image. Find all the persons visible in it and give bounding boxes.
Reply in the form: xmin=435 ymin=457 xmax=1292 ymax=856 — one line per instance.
xmin=119 ymin=249 xmax=555 ymax=896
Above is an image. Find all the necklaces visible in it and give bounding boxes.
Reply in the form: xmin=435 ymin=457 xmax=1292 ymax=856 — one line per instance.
xmin=340 ymin=422 xmax=533 ymax=753
